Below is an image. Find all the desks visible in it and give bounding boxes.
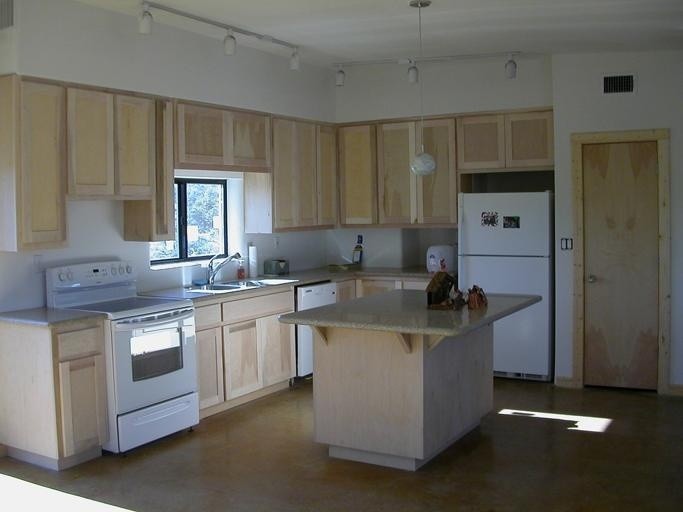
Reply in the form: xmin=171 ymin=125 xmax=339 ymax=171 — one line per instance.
xmin=278 ymin=295 xmax=542 ymax=472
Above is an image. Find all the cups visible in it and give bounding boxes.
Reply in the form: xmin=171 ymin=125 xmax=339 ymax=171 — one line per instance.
xmin=182 ymin=267 xmax=192 ymax=290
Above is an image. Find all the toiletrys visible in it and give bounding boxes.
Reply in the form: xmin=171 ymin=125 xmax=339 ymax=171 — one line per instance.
xmin=238 ymin=259 xmax=246 ymax=280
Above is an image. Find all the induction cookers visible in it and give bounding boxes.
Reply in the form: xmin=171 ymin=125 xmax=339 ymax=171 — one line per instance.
xmin=46 ymin=261 xmax=195 ymax=320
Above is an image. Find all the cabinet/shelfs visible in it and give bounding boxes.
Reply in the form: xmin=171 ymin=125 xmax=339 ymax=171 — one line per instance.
xmin=222 ymin=285 xmax=297 ymax=402
xmin=68 ymin=80 xmax=174 ymax=241
xmin=174 ymin=97 xmax=271 ymax=173
xmin=245 ymin=114 xmax=340 ymax=234
xmin=336 ymin=279 xmax=355 ymax=303
xmin=377 ymin=114 xmax=457 ymax=227
xmin=1 ymin=75 xmax=68 ymax=252
xmin=456 ymin=106 xmax=554 ymax=174
xmin=360 ymin=276 xmax=429 ymax=297
xmin=340 ymin=120 xmax=377 ymax=229
xmin=192 ymin=296 xmax=224 ymax=410
xmin=1 ymin=313 xmax=111 ymax=458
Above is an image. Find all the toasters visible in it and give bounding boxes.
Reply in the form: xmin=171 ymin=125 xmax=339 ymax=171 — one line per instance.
xmin=427 ymin=244 xmax=457 ymax=273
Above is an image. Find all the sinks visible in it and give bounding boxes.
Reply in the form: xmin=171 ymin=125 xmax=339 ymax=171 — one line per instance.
xmin=187 ymin=286 xmax=240 ymax=290
xmin=220 ymin=279 xmax=283 ymax=287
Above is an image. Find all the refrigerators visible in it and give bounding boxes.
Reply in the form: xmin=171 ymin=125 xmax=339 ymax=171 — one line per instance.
xmin=458 ymin=190 xmax=554 ymax=382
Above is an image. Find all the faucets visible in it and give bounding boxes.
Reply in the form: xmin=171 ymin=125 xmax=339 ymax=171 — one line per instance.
xmin=207 ymin=252 xmax=241 ymax=284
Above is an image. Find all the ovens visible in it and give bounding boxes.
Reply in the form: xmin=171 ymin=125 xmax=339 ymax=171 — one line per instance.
xmin=107 ymin=306 xmax=200 ymax=454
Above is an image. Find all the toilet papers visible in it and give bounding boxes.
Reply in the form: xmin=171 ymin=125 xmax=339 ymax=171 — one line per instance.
xmin=248 ymin=246 xmax=258 ymax=278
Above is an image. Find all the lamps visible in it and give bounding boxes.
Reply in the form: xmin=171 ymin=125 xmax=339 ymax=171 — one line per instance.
xmin=409 ymin=0 xmax=436 ymax=175
xmin=290 ymin=47 xmax=300 ymax=70
xmin=137 ymin=0 xmax=153 ymax=34
xmin=224 ymin=28 xmax=236 ymax=55
xmin=504 ymin=57 xmax=517 ymax=78
xmin=408 ymin=61 xmax=418 ymax=82
xmin=335 ymin=65 xmax=345 ymax=86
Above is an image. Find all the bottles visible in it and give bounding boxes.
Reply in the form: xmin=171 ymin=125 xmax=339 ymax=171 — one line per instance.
xmin=353 ymin=235 xmax=363 ymax=264
